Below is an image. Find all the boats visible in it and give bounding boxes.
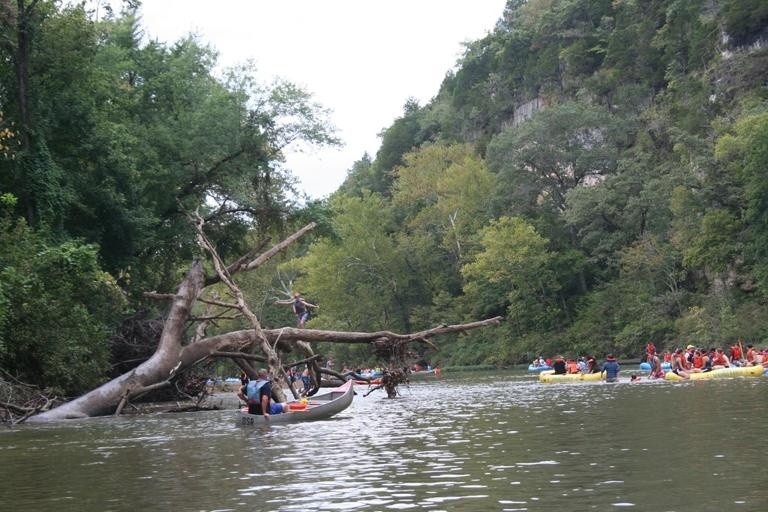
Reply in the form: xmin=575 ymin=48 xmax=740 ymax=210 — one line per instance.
xmin=527 ymin=353 xmax=552 ymax=371
xmin=541 ymin=368 xmax=608 ymax=383
xmin=236 ymin=379 xmax=354 ymax=429
xmin=666 ymin=364 xmax=762 ymax=382
xmin=640 ymin=362 xmax=670 ymax=370
xmin=342 ymin=367 xmax=441 ymax=383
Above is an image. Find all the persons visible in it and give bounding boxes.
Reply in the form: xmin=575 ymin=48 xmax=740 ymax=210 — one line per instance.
xmin=601 ymin=353 xmax=621 ymax=382
xmin=276 ymin=292 xmax=320 ymax=329
xmin=324 ymin=357 xmax=334 ymax=378
xmin=206 ymin=363 xmax=444 ymax=384
xmin=236 ymin=368 xmax=288 ymax=421
xmin=532 ymin=354 xmax=602 ymax=375
xmin=644 ymin=340 xmax=768 ymax=381
xmin=630 ymin=373 xmax=641 ymax=381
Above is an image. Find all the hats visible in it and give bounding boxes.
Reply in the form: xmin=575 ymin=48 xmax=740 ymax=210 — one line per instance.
xmin=607 ymin=353 xmax=616 ymax=360
xmin=687 ymin=344 xmax=694 ymax=350
